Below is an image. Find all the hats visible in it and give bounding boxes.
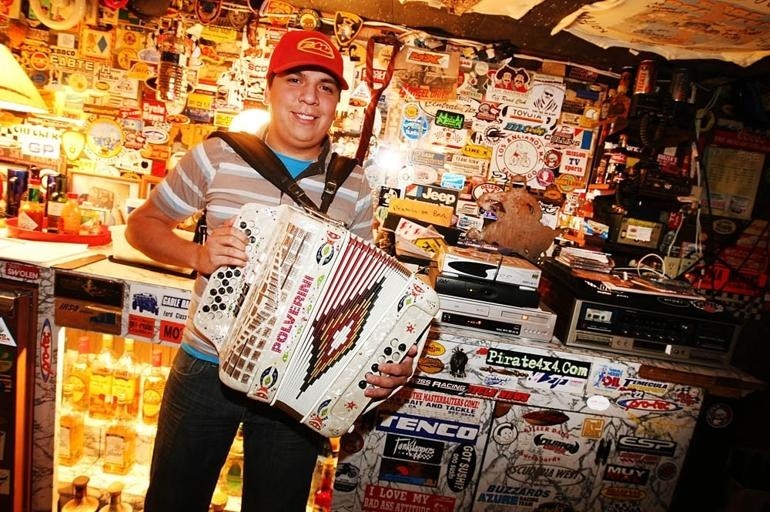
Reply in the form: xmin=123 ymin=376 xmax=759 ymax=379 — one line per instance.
xmin=266 ymin=29 xmax=349 ymax=89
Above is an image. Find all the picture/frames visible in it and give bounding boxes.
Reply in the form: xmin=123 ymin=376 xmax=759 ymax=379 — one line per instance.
xmin=142 ymin=174 xmax=163 ymax=199
xmin=29 ymin=0 xmax=87 ymax=31
xmin=65 ymin=167 xmax=141 ymax=224
xmin=617 ymin=217 xmax=663 ymax=249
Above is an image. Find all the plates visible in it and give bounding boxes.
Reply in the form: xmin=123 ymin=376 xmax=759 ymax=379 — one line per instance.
xmin=167 ymin=113 xmax=190 ymax=125
xmin=142 ymin=126 xmax=168 ymax=144
xmin=713 ymin=219 xmax=736 ymax=235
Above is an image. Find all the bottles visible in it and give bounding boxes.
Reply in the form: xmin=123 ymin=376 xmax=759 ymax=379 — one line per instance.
xmin=603 ymin=133 xmax=628 ymax=185
xmin=314 ymin=458 xmax=335 ymax=510
xmin=18 ymin=166 xmax=82 ymax=234
xmin=60 ymin=336 xmax=156 ymax=475
xmin=222 ymin=424 xmax=244 ymax=495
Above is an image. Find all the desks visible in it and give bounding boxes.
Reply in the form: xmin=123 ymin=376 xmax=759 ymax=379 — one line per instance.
xmin=0 ymin=225 xmax=770 ymax=509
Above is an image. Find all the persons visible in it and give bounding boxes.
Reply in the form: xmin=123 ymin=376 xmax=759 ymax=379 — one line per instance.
xmin=124 ymin=30 xmax=418 ymax=511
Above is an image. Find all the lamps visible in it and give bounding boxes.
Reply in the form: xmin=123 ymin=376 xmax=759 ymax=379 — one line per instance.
xmin=0 ymin=43 xmax=49 ymax=115
